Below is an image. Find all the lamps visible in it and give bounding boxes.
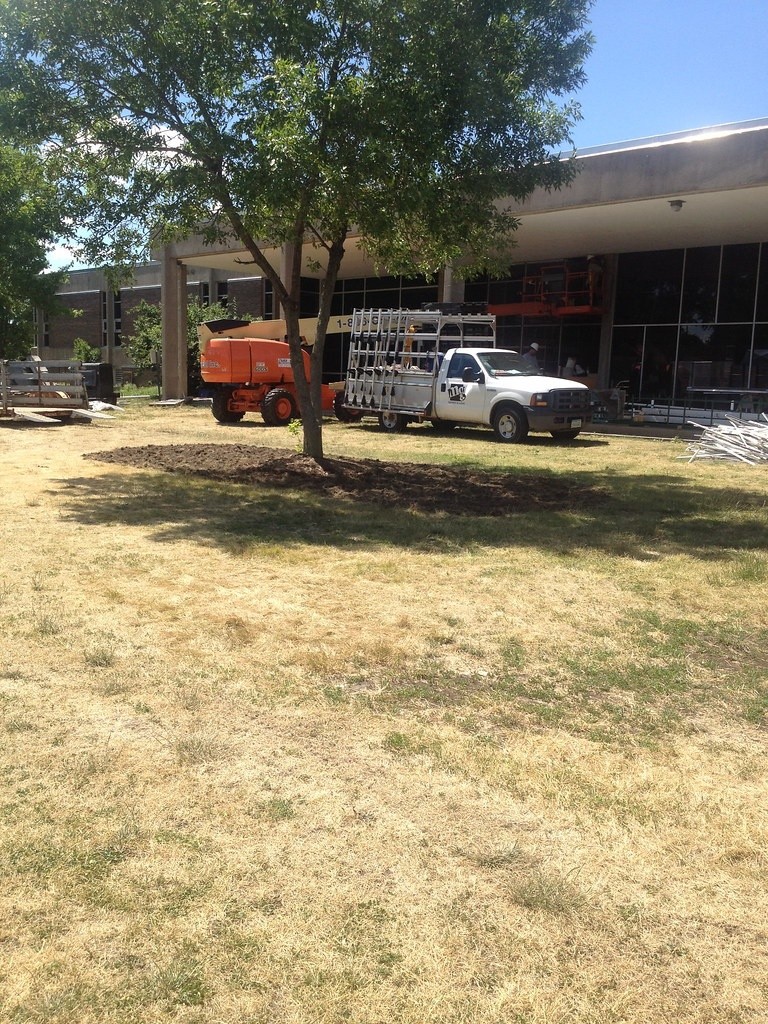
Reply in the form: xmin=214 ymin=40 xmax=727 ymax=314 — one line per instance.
xmin=668 ymin=199 xmax=686 ymax=211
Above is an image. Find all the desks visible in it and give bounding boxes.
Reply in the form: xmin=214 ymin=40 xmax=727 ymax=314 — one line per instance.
xmin=683 ymin=387 xmax=768 ymax=429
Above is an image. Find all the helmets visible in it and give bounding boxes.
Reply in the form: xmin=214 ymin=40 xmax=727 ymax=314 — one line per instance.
xmin=530 ymin=343 xmax=539 ymax=351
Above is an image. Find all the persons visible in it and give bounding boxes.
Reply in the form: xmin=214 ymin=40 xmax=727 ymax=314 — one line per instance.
xmin=523 ymin=343 xmax=539 ymax=369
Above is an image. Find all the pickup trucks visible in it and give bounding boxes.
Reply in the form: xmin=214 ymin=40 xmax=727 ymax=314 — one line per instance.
xmin=341 ymin=301 xmax=589 ymax=444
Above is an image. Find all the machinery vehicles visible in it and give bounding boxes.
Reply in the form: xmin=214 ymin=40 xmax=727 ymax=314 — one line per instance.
xmin=197 ymin=268 xmax=423 ymax=427
